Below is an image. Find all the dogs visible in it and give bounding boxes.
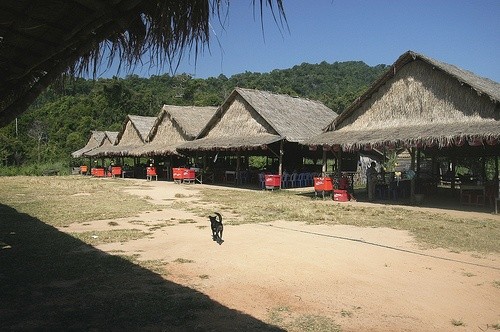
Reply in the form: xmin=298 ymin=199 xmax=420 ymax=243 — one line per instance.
xmin=208 ymin=212 xmax=224 ymax=240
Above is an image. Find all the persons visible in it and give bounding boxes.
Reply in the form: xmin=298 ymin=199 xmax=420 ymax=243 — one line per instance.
xmin=366 ymin=162 xmax=379 ymax=201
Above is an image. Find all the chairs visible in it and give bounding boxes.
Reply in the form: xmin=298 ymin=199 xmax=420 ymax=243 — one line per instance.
xmin=258 ymin=171 xmax=322 ymax=190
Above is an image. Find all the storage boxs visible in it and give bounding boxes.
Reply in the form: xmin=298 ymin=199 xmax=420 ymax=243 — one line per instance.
xmin=112 ymin=167 xmax=121 ymax=174
xmin=147 ymin=167 xmax=158 ymax=175
xmin=80 ymin=166 xmax=88 ymax=172
xmin=91 ymin=168 xmax=104 ymax=176
xmin=314 ymin=177 xmax=334 ymax=191
xmin=333 ymin=189 xmax=352 ymax=202
xmin=172 ymin=168 xmax=196 ymax=179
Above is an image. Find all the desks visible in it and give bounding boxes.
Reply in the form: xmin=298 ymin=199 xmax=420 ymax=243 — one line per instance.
xmin=459 ymin=184 xmax=486 ymax=210
xmin=224 ymin=170 xmax=247 ymax=185
